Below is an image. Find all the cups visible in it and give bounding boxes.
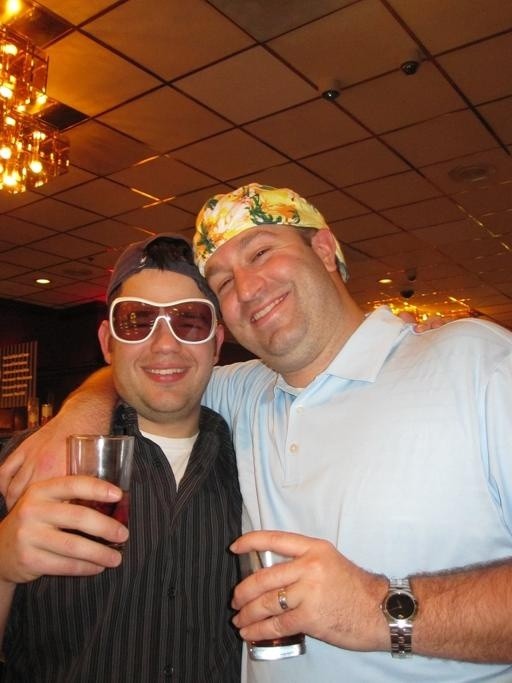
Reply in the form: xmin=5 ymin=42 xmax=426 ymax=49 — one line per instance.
xmin=225 ymin=533 xmax=312 ymax=662
xmin=63 ymin=431 xmax=135 ymax=560
xmin=28 ymin=396 xmax=40 ymax=430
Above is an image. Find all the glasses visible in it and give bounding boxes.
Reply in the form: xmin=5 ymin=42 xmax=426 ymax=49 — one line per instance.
xmin=109 ymin=297 xmax=217 ymax=346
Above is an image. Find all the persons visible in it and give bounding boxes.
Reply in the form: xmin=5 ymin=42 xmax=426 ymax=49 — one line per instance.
xmin=0 ymin=179 xmax=512 ymax=680
xmin=1 ymin=233 xmax=244 ymax=678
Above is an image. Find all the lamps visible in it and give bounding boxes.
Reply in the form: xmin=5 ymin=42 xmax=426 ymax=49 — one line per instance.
xmin=0 ymin=0 xmax=71 ymax=196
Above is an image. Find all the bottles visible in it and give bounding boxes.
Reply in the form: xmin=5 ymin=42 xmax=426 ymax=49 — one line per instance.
xmin=41 ymin=402 xmax=55 ymax=428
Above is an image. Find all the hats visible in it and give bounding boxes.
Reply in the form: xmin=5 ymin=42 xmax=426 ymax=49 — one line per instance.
xmin=193 ymin=182 xmax=351 ymax=283
xmin=105 ymin=233 xmax=221 ymax=319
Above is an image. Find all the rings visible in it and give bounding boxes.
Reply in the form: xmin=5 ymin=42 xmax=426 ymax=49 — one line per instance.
xmin=273 ymin=587 xmax=297 ymax=612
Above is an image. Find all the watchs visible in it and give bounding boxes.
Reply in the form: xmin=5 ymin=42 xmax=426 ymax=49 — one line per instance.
xmin=380 ymin=572 xmax=422 ymax=658
